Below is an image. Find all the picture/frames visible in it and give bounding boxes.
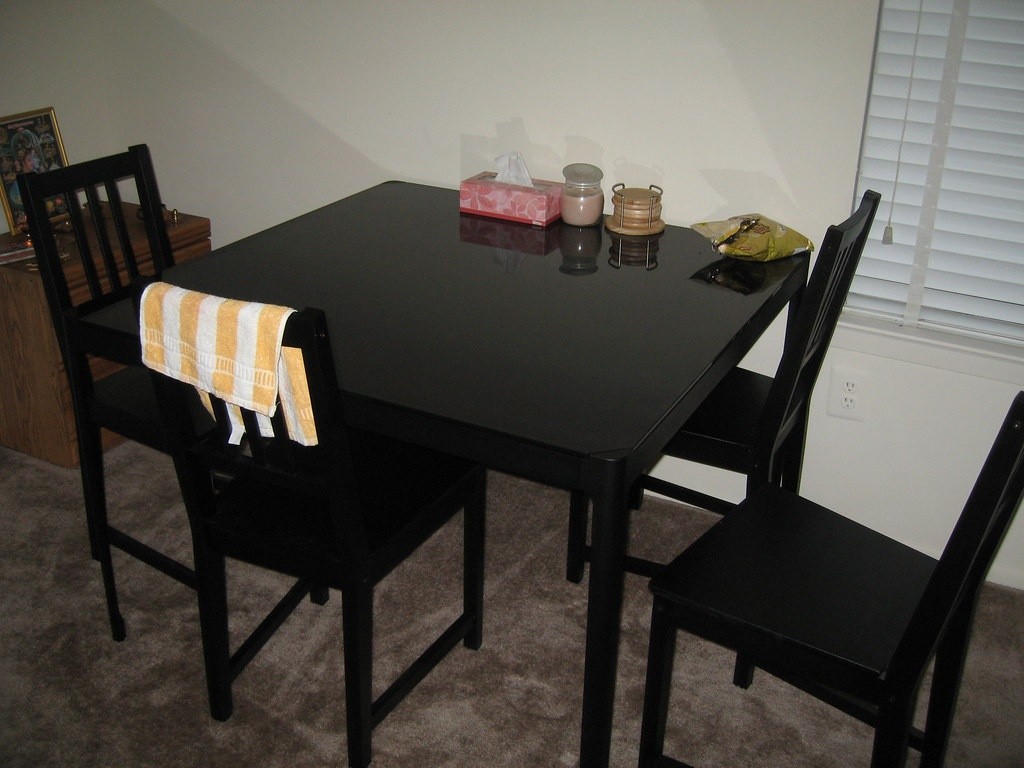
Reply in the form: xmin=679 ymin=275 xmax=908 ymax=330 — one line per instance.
xmin=0 ymin=106 xmax=67 ymax=237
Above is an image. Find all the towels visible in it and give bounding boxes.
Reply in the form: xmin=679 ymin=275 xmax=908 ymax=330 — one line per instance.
xmin=137 ymin=279 xmax=320 ymax=449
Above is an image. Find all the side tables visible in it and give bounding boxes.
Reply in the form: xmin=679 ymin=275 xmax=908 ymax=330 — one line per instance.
xmin=1 ymin=199 xmax=212 ymax=468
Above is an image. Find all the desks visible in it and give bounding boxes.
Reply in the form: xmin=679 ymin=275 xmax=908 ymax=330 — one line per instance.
xmin=81 ymin=180 xmax=811 ymax=768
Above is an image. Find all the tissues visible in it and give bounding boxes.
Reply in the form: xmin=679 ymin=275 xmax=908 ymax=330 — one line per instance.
xmin=459 ymin=150 xmax=566 ymax=226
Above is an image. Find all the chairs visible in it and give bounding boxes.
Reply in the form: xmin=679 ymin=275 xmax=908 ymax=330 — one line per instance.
xmin=638 ymin=391 xmax=1024 ymax=767
xmin=567 ymin=189 xmax=881 ymax=582
xmin=18 ymin=142 xmax=195 ymax=593
xmin=132 ymin=278 xmax=487 ymax=767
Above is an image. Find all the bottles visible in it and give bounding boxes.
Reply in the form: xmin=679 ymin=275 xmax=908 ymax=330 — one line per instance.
xmin=556 ymin=222 xmax=603 ymax=276
xmin=560 ymin=163 xmax=605 ymax=228
